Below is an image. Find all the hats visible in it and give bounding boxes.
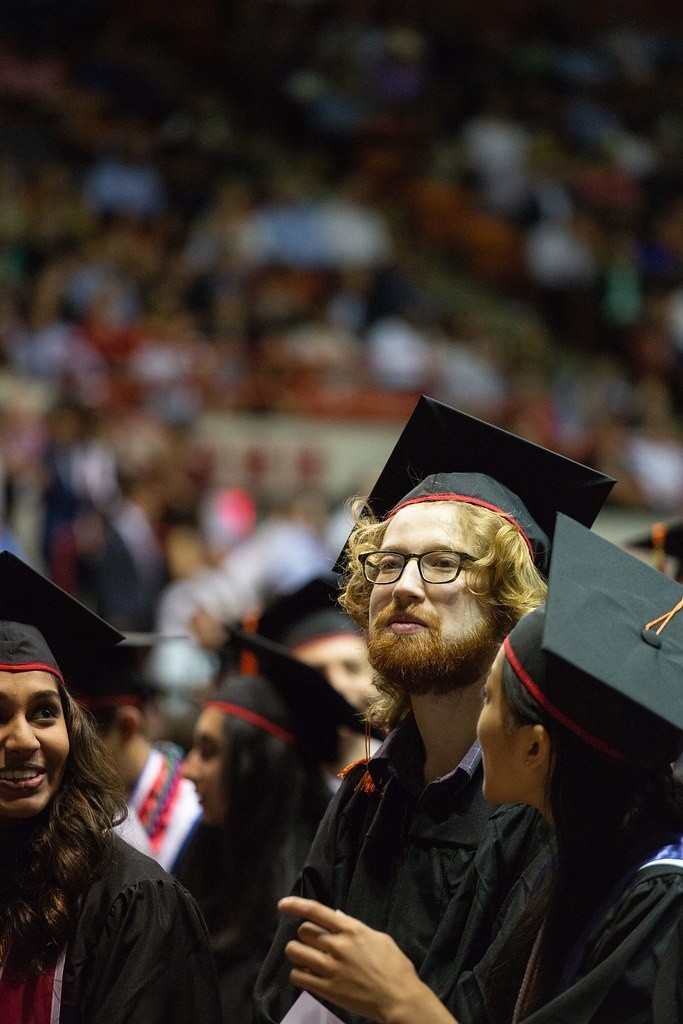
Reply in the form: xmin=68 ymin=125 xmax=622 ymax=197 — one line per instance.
xmin=504 ymin=511 xmax=682 ymax=766
xmin=198 ymin=621 xmax=387 ymax=764
xmin=67 ymin=639 xmax=171 ymax=703
xmin=256 ymin=575 xmax=368 ymax=649
xmin=0 ymin=550 xmax=125 ymax=685
xmin=330 ymin=392 xmax=620 ymax=576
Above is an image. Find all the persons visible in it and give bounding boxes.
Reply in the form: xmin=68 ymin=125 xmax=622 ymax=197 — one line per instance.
xmin=279 ymin=512 xmax=683 ymax=1024
xmin=256 ymin=577 xmax=382 ymax=791
xmin=163 ymin=629 xmax=357 ymax=1024
xmin=95 ymin=629 xmax=213 ymax=885
xmin=0 ymin=0 xmax=683 ymax=746
xmin=252 ymin=397 xmax=617 ymax=1024
xmin=0 ymin=551 xmax=223 ymax=1024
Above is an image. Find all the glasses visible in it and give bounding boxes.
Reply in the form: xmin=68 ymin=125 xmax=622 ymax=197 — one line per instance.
xmin=358 ymin=551 xmax=481 ymax=585
